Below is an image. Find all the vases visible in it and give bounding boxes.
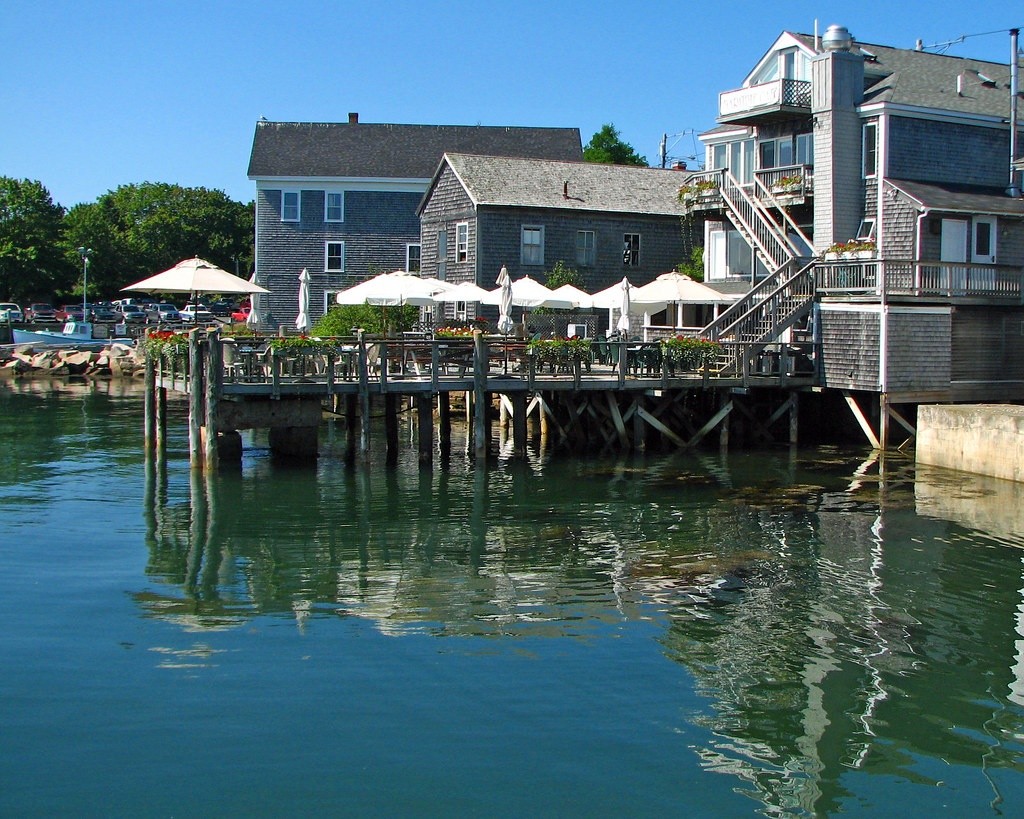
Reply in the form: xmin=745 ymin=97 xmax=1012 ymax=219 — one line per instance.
xmin=683 ymin=188 xmax=719 ymax=200
xmin=772 ymin=183 xmax=802 ymax=193
xmin=825 ymin=250 xmax=877 ymax=261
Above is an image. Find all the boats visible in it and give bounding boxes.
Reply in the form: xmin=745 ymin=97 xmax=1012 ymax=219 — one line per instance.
xmin=14 ymin=323 xmax=132 ymax=349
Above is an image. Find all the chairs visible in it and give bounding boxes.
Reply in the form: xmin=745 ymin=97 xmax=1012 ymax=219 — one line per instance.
xmin=220 ymin=334 xmax=666 ymax=384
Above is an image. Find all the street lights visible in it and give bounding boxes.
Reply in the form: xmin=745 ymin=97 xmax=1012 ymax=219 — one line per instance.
xmin=78 ymin=246 xmax=91 ymax=322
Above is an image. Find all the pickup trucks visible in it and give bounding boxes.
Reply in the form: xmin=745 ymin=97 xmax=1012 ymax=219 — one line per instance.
xmin=145 ymin=304 xmax=181 ymax=324
xmin=24 ymin=304 xmax=57 ymax=323
xmin=178 ymin=304 xmax=213 ymax=322
xmin=115 ymin=305 xmax=147 ymax=323
xmin=54 ymin=305 xmax=84 ymax=322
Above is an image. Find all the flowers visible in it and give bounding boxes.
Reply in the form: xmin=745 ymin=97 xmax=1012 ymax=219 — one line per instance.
xmin=677 ymin=180 xmax=717 ymax=204
xmin=827 ymin=240 xmax=873 ymax=253
xmin=771 ymin=175 xmax=803 ymax=193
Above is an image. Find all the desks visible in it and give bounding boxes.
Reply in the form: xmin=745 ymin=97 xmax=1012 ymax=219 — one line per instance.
xmin=288 ymin=351 xmax=319 ymax=383
xmin=626 ymin=348 xmax=658 ymax=378
xmin=590 ymin=341 xmax=659 ymax=377
xmin=224 ymin=340 xmax=265 ymax=378
xmin=341 ymin=349 xmax=359 ymax=382
xmin=238 ymin=350 xmax=265 ymax=383
xmin=385 ymin=347 xmax=417 ymax=380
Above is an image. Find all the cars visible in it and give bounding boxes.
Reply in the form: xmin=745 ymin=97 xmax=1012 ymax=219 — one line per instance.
xmin=0 ymin=303 xmax=23 ymax=322
xmin=91 ymin=307 xmax=116 ymax=323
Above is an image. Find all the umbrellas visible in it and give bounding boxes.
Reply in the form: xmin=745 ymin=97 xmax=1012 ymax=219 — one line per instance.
xmin=295 ymin=267 xmax=312 ymax=337
xmin=616 ymin=276 xmax=632 ymax=335
xmin=119 ymin=254 xmax=272 ymax=328
xmin=495 ymin=264 xmax=512 ymax=286
xmin=336 ymin=267 xmax=736 ymax=330
xmin=246 ymin=272 xmax=263 ymax=339
xmin=497 ymin=274 xmax=514 ymax=376
